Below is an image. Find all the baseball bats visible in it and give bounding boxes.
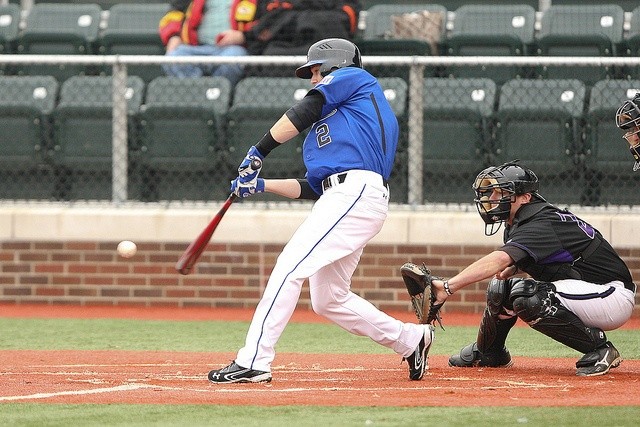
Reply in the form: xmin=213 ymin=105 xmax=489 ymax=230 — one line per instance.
xmin=176 ymin=159 xmax=261 ymax=274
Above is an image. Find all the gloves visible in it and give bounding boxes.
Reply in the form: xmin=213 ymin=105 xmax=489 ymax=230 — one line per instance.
xmin=237 ymin=145 xmax=263 ymax=185
xmin=230 ymin=174 xmax=266 ymax=197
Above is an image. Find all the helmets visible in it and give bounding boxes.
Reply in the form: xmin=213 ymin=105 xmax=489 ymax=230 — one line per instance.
xmin=294 ymin=36 xmax=363 ymax=79
xmin=614 ymin=94 xmax=639 ymax=176
xmin=473 ymin=161 xmax=539 ymax=237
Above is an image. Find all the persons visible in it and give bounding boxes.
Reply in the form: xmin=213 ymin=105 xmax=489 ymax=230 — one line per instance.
xmin=612 ymin=89 xmax=640 ymax=173
xmin=214 ymin=0 xmax=361 ymax=59
xmin=158 ymin=0 xmax=256 ymax=89
xmin=400 ymin=161 xmax=637 ymax=379
xmin=207 ymin=38 xmax=436 ymax=387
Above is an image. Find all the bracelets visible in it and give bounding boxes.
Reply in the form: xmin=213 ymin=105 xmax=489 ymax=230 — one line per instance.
xmin=444 ymin=280 xmax=453 ymax=295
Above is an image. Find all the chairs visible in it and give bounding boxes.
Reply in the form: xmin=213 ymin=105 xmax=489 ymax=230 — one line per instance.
xmin=96 ymin=1 xmax=177 ymax=81
xmin=0 ymin=3 xmax=20 ymax=74
xmin=24 ymin=0 xmax=103 ymax=76
xmin=583 ymin=80 xmax=640 ymax=205
xmin=493 ymin=75 xmax=586 ymax=202
xmin=450 ymin=0 xmax=536 ymax=74
xmin=409 ymin=76 xmax=497 ymax=203
xmin=529 ymin=1 xmax=622 ymax=79
xmin=373 ymin=76 xmax=408 ymax=202
xmin=358 ymin=0 xmax=446 ymax=78
xmin=614 ymin=0 xmax=640 ymax=60
xmin=225 ymin=73 xmax=313 ymax=202
xmin=52 ymin=73 xmax=140 ymax=200
xmin=0 ymin=71 xmax=57 ymax=199
xmin=135 ymin=74 xmax=230 ymax=199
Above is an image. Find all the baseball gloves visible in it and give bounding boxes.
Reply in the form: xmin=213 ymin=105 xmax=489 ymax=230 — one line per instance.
xmin=400 ymin=262 xmax=444 ymax=330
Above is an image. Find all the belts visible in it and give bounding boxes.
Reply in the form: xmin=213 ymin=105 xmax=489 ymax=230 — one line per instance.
xmin=619 ymin=281 xmax=637 ymax=293
xmin=319 ymin=173 xmax=388 ymax=190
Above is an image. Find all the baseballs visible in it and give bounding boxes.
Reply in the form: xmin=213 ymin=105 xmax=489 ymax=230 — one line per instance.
xmin=117 ymin=241 xmax=137 ymax=258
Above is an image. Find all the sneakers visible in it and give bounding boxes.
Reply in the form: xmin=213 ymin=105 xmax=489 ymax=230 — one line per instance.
xmin=400 ymin=323 xmax=434 ymax=381
xmin=448 ymin=345 xmax=514 ymax=369
xmin=207 ymin=361 xmax=273 ymax=384
xmin=575 ymin=342 xmax=622 ymax=378
